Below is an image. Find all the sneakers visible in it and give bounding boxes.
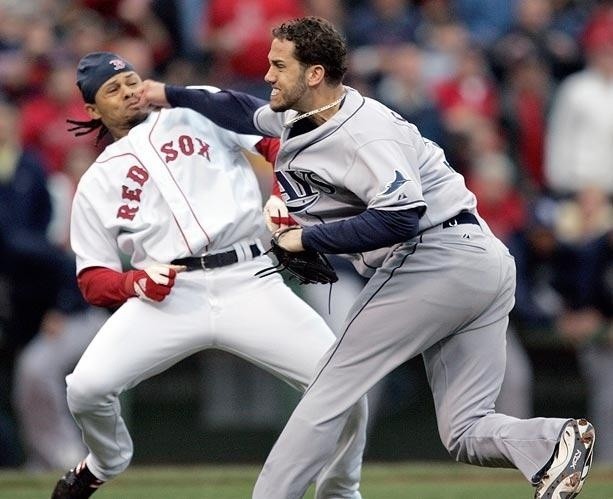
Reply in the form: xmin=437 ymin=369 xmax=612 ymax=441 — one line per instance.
xmin=51 ymin=460 xmax=104 ymax=499
xmin=535 ymin=419 xmax=595 ymax=499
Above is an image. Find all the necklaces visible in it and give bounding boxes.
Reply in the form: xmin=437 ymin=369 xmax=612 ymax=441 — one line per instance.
xmin=282 ymin=92 xmax=345 ymax=128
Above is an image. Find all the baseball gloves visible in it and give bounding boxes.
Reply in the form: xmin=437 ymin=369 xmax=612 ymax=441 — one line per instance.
xmin=254 ymin=226 xmax=338 ymax=315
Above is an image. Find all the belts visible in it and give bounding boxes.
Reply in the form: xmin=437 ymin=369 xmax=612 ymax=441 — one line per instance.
xmin=444 ymin=209 xmax=479 ymax=227
xmin=172 ymin=244 xmax=260 ymax=269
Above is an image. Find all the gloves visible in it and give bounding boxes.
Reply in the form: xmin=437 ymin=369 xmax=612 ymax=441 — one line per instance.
xmin=263 ymin=194 xmax=301 ymax=233
xmin=126 ymin=267 xmax=176 ymax=303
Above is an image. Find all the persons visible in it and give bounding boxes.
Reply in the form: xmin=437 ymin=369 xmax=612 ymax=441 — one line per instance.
xmin=138 ymin=16 xmax=596 ymax=497
xmin=1 ymin=0 xmax=613 ymax=473
xmin=51 ymin=52 xmax=367 ymax=498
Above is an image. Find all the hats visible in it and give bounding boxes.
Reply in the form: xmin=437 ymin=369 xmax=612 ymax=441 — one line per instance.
xmin=76 ymin=52 xmax=137 ymax=102
